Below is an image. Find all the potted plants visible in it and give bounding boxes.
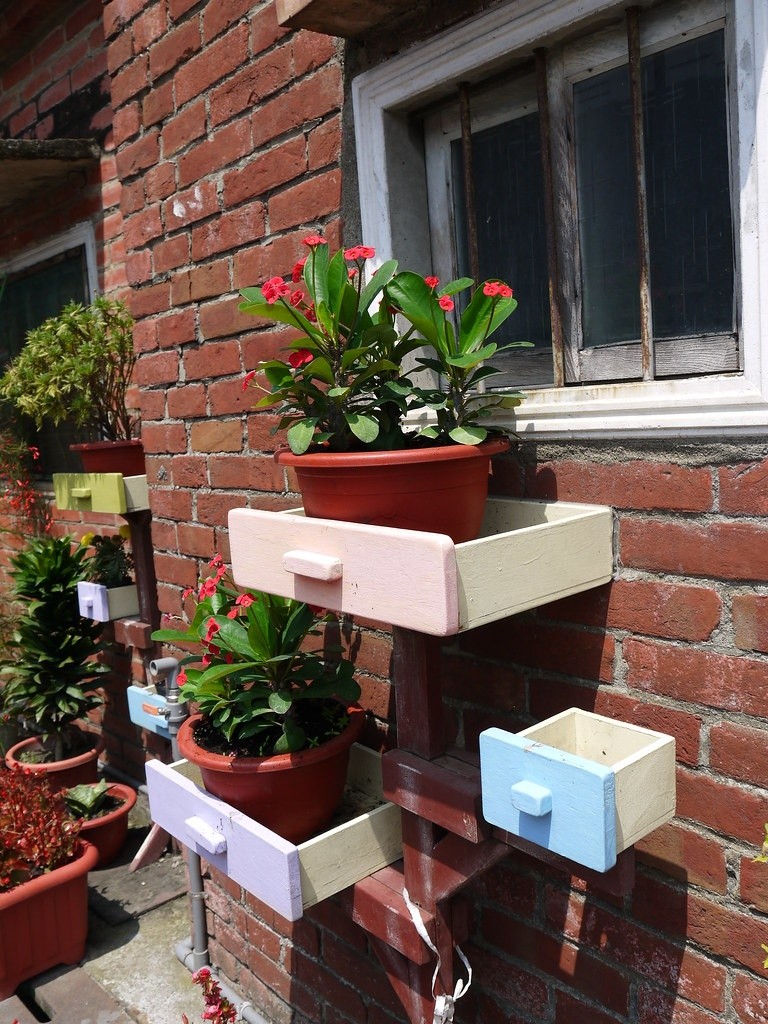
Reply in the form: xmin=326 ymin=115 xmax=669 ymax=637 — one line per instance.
xmin=0 ymin=537 xmax=115 ymax=794
xmin=77 ymin=535 xmax=141 ymax=622
xmin=53 ymin=778 xmax=137 ymax=871
xmin=1 ymin=290 xmax=145 ymax=477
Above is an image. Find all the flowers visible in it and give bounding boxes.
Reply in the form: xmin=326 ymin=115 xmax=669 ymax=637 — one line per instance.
xmin=0 ymin=763 xmax=85 ymax=887
xmin=238 ymin=233 xmax=532 ymax=448
xmin=150 ymin=556 xmax=360 ymax=755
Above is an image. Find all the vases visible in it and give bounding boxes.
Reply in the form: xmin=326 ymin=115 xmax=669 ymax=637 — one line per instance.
xmin=271 ymin=433 xmax=507 ymax=543
xmin=0 ymin=837 xmax=97 ymax=1000
xmin=180 ymin=706 xmax=360 ymax=843
